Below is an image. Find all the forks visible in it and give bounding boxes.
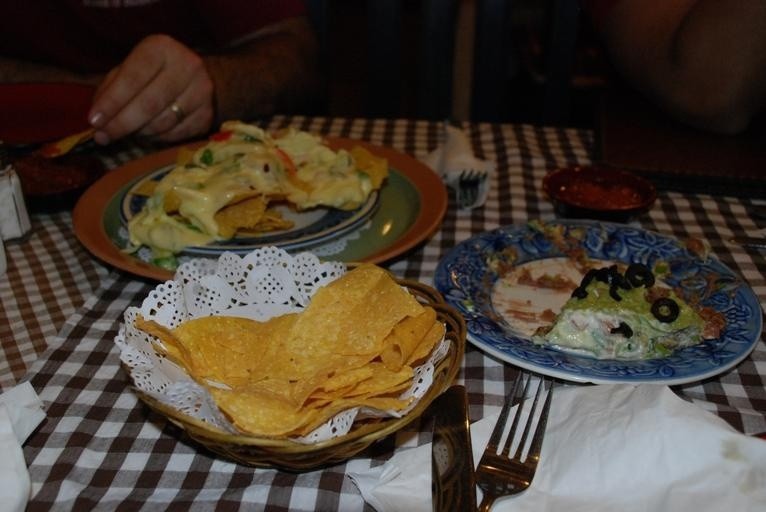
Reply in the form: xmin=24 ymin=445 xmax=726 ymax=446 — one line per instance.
xmin=476 ymin=370 xmax=556 ymax=507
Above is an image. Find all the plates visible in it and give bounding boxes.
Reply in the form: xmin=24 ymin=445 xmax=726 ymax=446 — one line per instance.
xmin=72 ymin=133 xmax=450 ymax=284
xmin=118 ymin=161 xmax=381 ymax=257
xmin=432 ymin=219 xmax=762 ymax=387
xmin=0 ymin=80 xmax=93 ymax=155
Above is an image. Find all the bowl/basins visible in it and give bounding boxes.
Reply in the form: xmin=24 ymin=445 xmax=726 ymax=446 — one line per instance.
xmin=542 ymin=162 xmax=658 ymax=222
xmin=0 ymin=143 xmax=104 ymax=206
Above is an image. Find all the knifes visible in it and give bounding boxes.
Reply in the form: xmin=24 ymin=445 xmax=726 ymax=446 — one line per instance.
xmin=431 ymin=384 xmax=479 ymax=508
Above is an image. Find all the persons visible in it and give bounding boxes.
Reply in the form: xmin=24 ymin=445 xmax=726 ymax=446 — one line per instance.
xmin=2 ymin=1 xmax=319 ymax=151
xmin=608 ymin=0 xmax=766 ymax=135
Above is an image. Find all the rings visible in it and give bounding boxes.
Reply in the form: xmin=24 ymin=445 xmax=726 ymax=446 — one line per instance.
xmin=169 ymin=102 xmax=186 ymax=122
